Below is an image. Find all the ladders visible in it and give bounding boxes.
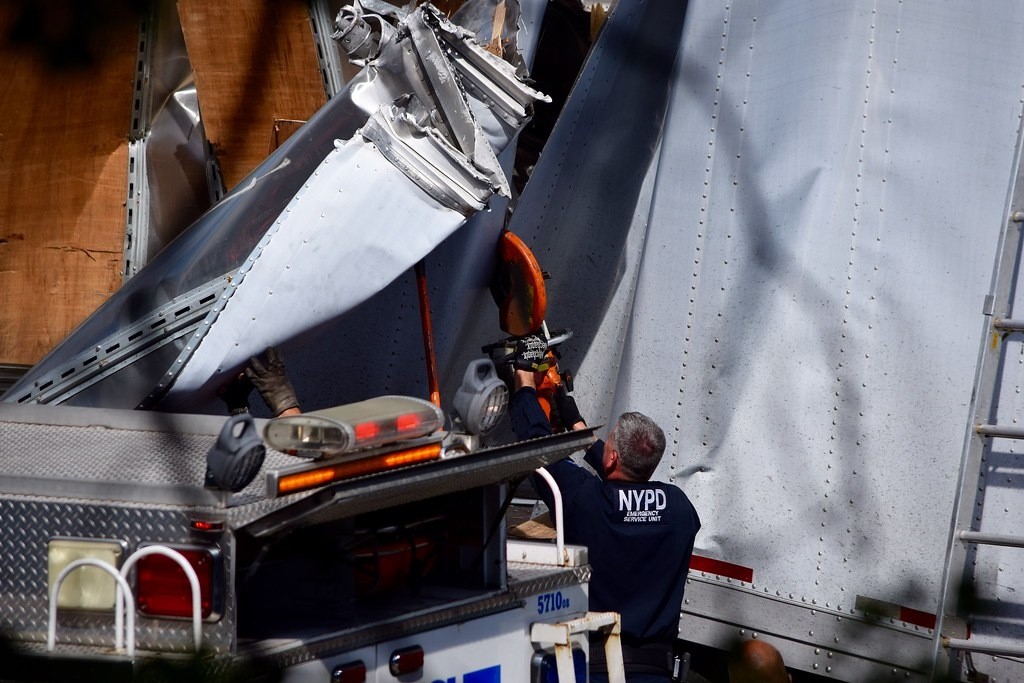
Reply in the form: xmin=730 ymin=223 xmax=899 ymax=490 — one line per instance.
xmin=928 ymin=129 xmax=1023 ymax=682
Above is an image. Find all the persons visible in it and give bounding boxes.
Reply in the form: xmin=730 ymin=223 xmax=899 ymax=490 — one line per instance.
xmin=99 ymin=247 xmax=301 ymax=419
xmin=505 ymin=333 xmax=701 ymax=683
xmin=725 ymin=639 xmax=795 ymax=683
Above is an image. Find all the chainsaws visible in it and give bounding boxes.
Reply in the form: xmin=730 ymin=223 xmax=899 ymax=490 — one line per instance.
xmin=473 ymin=232 xmax=584 ymax=426
